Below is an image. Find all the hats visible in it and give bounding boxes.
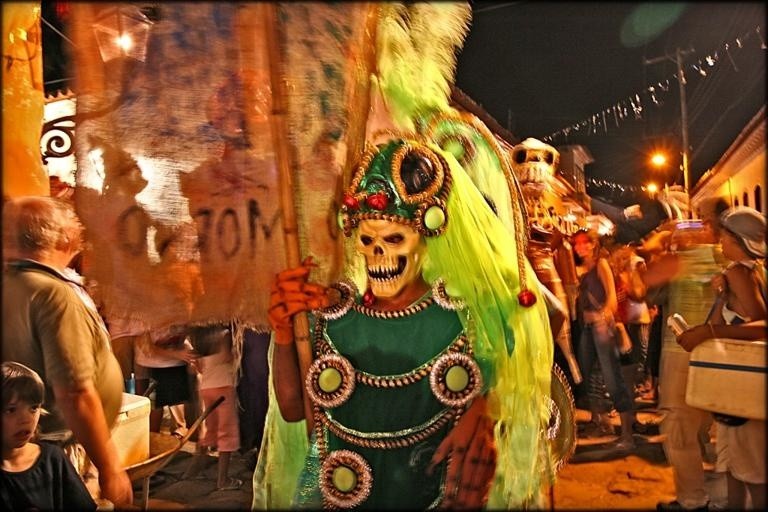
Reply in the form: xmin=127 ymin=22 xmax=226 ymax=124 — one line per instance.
xmin=339 ymin=137 xmax=452 ymax=237
xmin=721 ymin=207 xmax=767 ymax=259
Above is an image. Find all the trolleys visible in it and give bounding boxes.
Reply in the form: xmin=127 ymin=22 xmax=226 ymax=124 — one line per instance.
xmin=121 ymin=379 xmax=225 ymax=512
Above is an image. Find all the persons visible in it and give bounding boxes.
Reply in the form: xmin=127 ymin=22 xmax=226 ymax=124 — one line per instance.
xmin=542 ymin=195 xmax=768 ymax=510
xmin=0 ymin=360 xmax=102 ymax=511
xmin=265 ymin=138 xmax=500 ymax=512
xmin=101 ymin=318 xmax=280 ymax=490
xmin=0 ymin=195 xmax=135 ymax=509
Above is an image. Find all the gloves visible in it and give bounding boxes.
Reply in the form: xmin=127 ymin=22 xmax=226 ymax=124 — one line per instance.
xmin=266 ymin=257 xmax=330 ymax=344
xmin=428 ymin=397 xmax=498 ymax=507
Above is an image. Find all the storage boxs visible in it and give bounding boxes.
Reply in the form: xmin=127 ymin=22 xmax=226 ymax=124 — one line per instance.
xmin=684 ymin=337 xmax=768 ymax=421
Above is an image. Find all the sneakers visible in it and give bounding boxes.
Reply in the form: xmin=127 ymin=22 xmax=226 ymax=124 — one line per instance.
xmin=657 ymin=499 xmax=708 ymax=512
xmin=586 ymin=417 xmax=658 ymax=454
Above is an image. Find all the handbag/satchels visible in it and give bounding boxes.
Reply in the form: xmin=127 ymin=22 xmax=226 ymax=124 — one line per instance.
xmin=613 ymin=321 xmax=632 ymax=355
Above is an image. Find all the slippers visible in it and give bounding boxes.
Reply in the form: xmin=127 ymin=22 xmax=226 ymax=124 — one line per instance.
xmin=217 ymin=477 xmax=242 ymax=492
